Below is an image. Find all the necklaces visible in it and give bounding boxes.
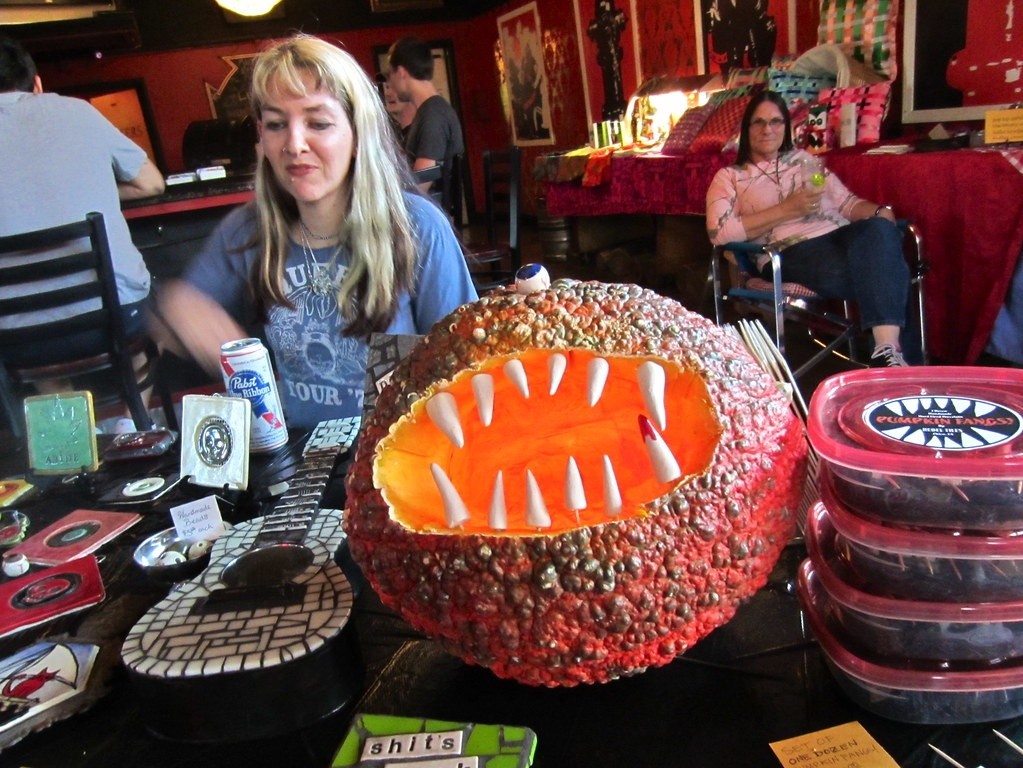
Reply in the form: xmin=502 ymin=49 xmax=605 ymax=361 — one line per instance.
xmin=747 ymin=154 xmax=784 ymax=204
xmin=298 ymin=215 xmax=345 ymax=297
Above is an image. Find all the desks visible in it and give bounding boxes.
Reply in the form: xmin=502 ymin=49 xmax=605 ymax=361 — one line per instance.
xmin=120 ymin=169 xmax=262 ymax=423
xmin=0 ymin=379 xmax=1023 ymax=768
xmin=534 ymin=145 xmax=1023 ymax=370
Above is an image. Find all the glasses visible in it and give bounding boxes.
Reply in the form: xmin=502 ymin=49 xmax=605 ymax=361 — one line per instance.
xmin=748 ymin=115 xmax=787 ymax=130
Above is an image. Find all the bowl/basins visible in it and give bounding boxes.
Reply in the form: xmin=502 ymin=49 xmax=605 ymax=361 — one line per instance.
xmin=134 ymin=522 xmax=233 ymax=578
xmin=795 ymin=363 xmax=1023 ymax=726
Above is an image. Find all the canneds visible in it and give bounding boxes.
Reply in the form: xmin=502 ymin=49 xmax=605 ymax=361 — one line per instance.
xmin=219 ymin=337 xmax=289 ymax=453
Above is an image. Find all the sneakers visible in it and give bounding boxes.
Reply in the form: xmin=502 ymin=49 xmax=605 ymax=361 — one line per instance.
xmin=871 ymin=345 xmax=907 ymax=367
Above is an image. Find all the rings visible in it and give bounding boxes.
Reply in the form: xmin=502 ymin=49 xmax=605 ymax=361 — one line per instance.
xmin=813 ymin=203 xmax=816 ymax=208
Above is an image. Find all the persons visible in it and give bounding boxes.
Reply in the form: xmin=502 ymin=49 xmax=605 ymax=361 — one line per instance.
xmin=518 ymin=39 xmax=542 ymax=137
xmin=0 ymin=30 xmax=166 ymax=435
xmin=387 ymin=36 xmax=463 ymax=223
xmin=167 ymin=25 xmax=480 ymax=428
xmin=705 ymin=90 xmax=910 ymax=368
xmin=181 ymin=78 xmax=260 ymax=169
xmin=375 ymin=71 xmax=418 ymax=151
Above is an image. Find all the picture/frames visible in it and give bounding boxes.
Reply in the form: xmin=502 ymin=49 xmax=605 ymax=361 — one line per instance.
xmin=629 ymin=0 xmax=705 ymax=88
xmin=497 ymin=1 xmax=556 ymax=147
xmin=900 ymin=0 xmax=1023 ymax=124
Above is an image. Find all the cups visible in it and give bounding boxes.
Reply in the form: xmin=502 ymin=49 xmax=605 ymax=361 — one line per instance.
xmin=800 ymin=159 xmax=824 ymax=193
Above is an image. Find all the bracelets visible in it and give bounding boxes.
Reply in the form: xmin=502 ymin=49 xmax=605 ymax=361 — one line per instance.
xmin=875 ymin=205 xmax=885 ymax=216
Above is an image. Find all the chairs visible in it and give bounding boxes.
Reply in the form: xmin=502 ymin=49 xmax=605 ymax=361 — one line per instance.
xmin=0 ymin=149 xmax=525 ymax=478
xmin=707 ymin=220 xmax=929 ymax=375
